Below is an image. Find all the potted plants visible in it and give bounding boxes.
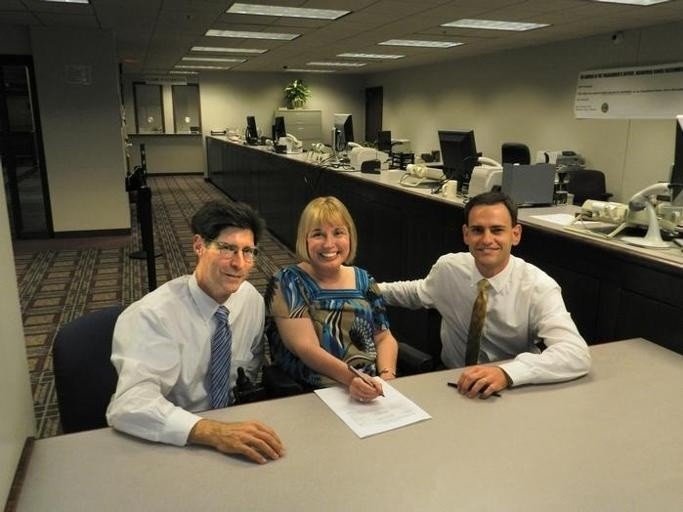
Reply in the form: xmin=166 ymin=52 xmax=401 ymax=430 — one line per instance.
xmin=281 ymin=79 xmax=313 ymax=109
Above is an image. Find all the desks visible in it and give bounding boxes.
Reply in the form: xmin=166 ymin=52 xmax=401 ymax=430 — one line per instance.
xmin=5 ymin=336 xmax=682 ymax=512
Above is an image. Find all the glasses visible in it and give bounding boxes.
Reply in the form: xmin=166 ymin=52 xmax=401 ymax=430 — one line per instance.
xmin=204 ymin=237 xmax=261 ymax=265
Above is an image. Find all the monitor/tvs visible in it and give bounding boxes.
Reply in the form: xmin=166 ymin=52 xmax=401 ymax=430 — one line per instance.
xmin=272 ymin=116 xmax=286 ymax=140
xmin=245 ymin=116 xmax=258 ymax=144
xmin=437 ymin=129 xmax=477 ymax=180
xmin=333 ymin=112 xmax=354 ymax=152
xmin=667 ymin=113 xmax=683 ymax=197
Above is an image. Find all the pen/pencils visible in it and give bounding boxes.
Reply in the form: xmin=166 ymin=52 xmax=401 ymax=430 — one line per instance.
xmin=448 ymin=383 xmax=501 ymax=397
xmin=348 ymin=365 xmax=385 ymax=397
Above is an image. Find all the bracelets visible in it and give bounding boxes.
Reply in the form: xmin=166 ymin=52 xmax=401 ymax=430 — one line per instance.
xmin=377 ymin=367 xmax=397 ymax=377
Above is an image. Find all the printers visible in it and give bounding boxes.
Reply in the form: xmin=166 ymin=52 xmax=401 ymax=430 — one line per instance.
xmin=536 ymin=148 xmax=586 ymax=182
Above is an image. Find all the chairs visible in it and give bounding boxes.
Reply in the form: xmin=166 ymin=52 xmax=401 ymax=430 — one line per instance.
xmin=51 ymin=306 xmax=127 ymax=433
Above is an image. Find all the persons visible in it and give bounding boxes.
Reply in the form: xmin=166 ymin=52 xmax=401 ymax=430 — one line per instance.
xmin=102 ymin=199 xmax=287 ymax=464
xmin=265 ymin=195 xmax=400 ymax=404
xmin=375 ymin=191 xmax=595 ymax=399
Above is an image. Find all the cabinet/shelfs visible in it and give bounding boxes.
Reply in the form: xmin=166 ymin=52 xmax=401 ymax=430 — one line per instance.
xmin=274 ymin=110 xmax=322 ymax=150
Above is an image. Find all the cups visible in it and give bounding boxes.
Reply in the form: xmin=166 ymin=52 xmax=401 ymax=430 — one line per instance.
xmin=439 ymin=179 xmax=458 ymax=199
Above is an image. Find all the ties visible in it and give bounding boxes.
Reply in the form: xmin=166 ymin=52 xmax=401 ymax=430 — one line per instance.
xmin=208 ymin=304 xmax=234 ymax=410
xmin=464 ymin=277 xmax=492 ymax=367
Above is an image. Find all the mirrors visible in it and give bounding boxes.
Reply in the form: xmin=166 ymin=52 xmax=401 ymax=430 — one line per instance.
xmin=171 ymin=83 xmax=201 ymax=133
xmin=132 ymin=81 xmax=166 ymax=133
